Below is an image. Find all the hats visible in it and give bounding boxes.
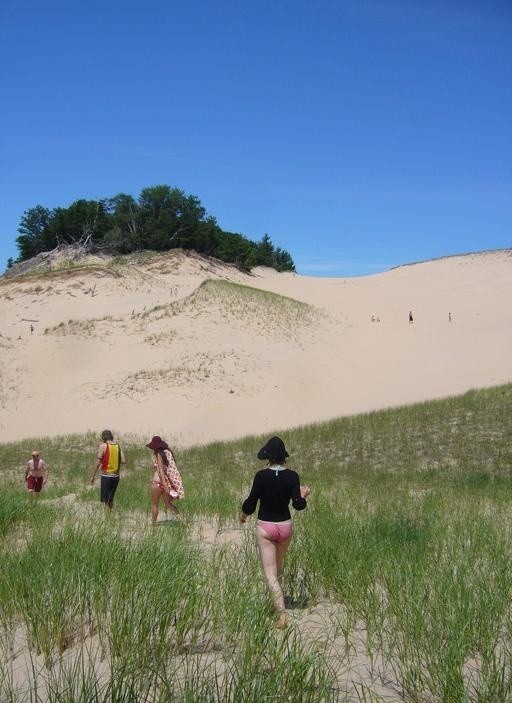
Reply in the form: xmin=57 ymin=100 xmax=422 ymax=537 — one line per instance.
xmin=146 ymin=436 xmax=168 ymax=449
xmin=102 ymin=430 xmax=112 ymax=438
xmin=258 ymin=436 xmax=289 ymax=459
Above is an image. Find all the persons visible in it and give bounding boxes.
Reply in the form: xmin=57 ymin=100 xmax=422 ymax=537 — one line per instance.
xmin=240 ymin=437 xmax=310 ymax=627
xmin=23 ymin=450 xmax=49 ymax=503
xmin=90 ymin=430 xmax=127 ymax=513
xmin=146 ymin=436 xmax=185 ymax=527
